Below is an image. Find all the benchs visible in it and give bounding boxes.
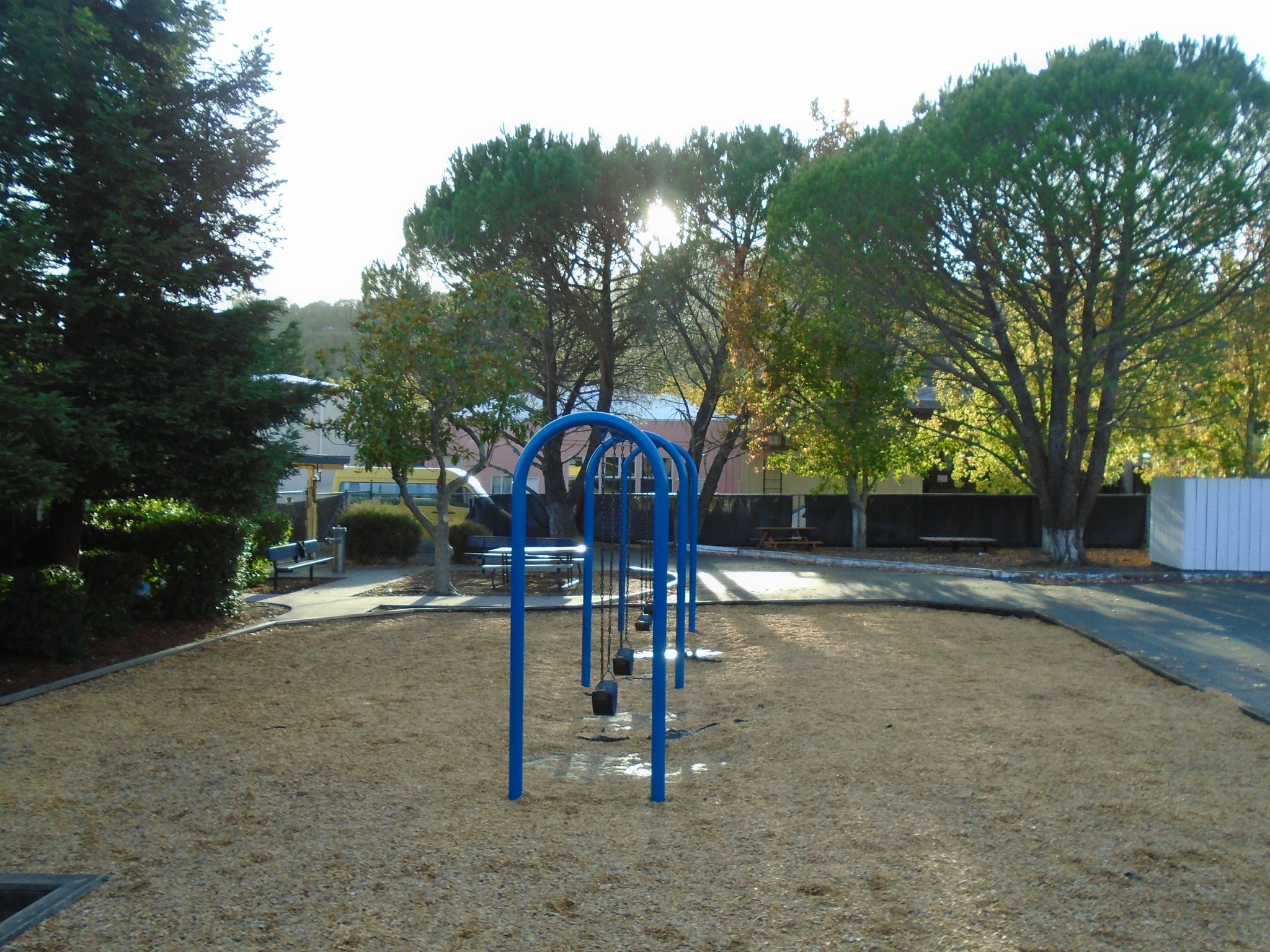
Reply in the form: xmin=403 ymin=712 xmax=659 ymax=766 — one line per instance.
xmin=763 ymin=541 xmax=824 ymax=554
xmin=749 ymin=536 xmax=802 ymax=550
xmin=920 ymin=537 xmax=999 ymax=554
xmin=464 ymin=534 xmax=578 ymax=575
xmin=480 ymin=564 xmax=575 ymax=592
xmin=268 ymin=539 xmax=335 ymax=590
xmin=502 ymin=557 xmax=585 ymax=584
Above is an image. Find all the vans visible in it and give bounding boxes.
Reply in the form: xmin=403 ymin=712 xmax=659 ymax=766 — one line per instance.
xmin=332 ymin=465 xmax=513 ymax=542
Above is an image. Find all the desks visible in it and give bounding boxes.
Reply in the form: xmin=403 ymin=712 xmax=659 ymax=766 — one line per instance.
xmin=754 ymin=526 xmax=818 ymax=551
xmin=488 ymin=545 xmax=587 ymax=587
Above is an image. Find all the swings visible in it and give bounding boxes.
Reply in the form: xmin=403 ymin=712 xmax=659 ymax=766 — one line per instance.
xmin=591 ymin=425 xmax=620 ymax=717
xmin=641 ymin=460 xmax=655 ymax=616
xmin=633 ymin=450 xmax=654 ymax=632
xmin=612 ymin=434 xmax=636 ymax=677
